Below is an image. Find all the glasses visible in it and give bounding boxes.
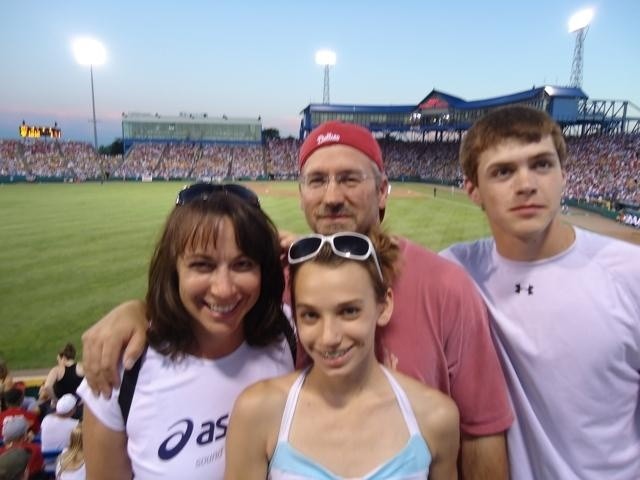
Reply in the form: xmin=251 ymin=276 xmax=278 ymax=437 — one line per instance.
xmin=287 ymin=231 xmax=385 ymax=285
xmin=175 ymin=183 xmax=259 ymax=208
xmin=298 ymin=169 xmax=374 ymax=190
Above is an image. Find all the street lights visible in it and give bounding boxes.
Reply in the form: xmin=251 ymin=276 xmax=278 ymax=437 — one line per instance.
xmin=314 ymin=48 xmax=339 ymax=105
xmin=563 ymin=7 xmax=595 ymax=89
xmin=71 ymin=34 xmax=109 ymax=154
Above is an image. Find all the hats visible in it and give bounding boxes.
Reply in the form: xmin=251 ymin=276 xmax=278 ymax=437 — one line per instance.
xmin=55 ymin=392 xmax=79 ymax=416
xmin=297 ymin=119 xmax=384 ymax=174
xmin=1 ymin=448 xmax=33 ymax=480
xmin=2 ymin=416 xmax=30 ymax=445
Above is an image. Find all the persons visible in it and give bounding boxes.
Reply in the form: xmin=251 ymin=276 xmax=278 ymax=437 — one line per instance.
xmin=0 ymin=135 xmax=304 ymax=183
xmin=561 ymin=129 xmax=640 ymax=230
xmin=76 ymin=182 xmax=297 ymax=480
xmin=435 ymin=102 xmax=639 ymax=479
xmin=222 ymin=231 xmax=460 ymax=480
xmin=0 ymin=341 xmax=86 ymax=480
xmin=372 ymin=115 xmax=466 ymax=185
xmin=80 ymin=118 xmax=514 ymax=480
